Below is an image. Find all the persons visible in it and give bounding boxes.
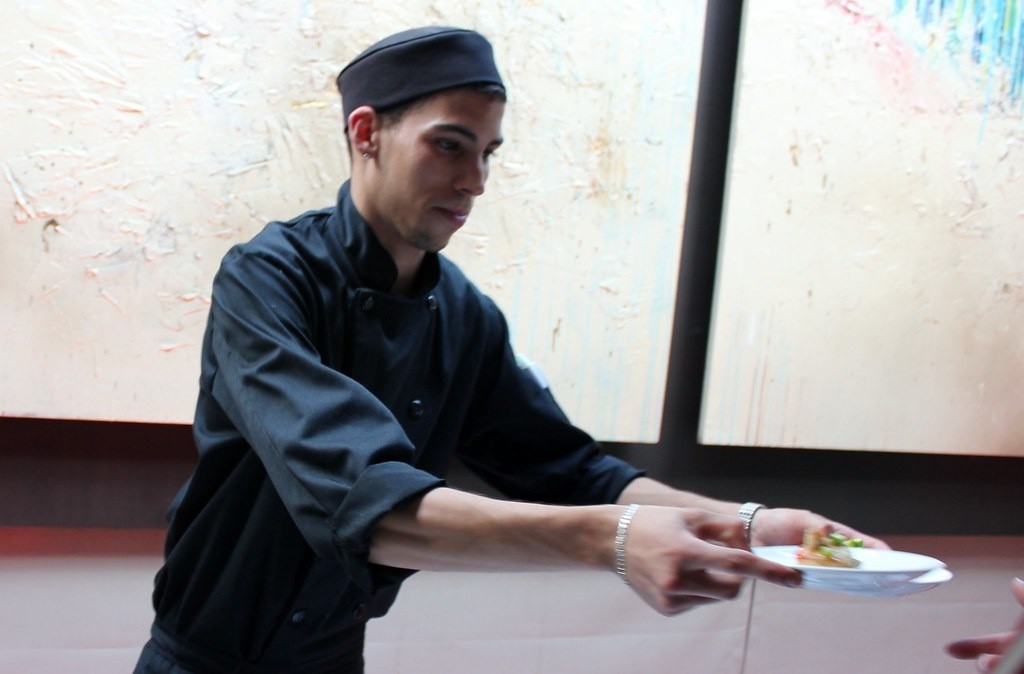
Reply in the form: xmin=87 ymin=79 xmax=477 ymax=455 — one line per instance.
xmin=131 ymin=25 xmax=894 ymax=674
xmin=941 ymin=577 xmax=1024 ymax=674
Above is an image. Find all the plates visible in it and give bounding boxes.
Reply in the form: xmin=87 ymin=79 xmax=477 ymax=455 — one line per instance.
xmin=750 ymin=547 xmax=941 ymax=592
xmin=889 ymin=566 xmax=958 ymax=593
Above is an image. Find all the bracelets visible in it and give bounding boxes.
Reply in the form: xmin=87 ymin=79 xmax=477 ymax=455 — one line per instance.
xmin=736 ymin=500 xmax=766 ymax=545
xmin=614 ymin=503 xmax=641 ymax=586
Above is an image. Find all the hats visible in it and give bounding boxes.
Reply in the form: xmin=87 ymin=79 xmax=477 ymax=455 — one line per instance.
xmin=336 ymin=26 xmax=506 ymax=133
